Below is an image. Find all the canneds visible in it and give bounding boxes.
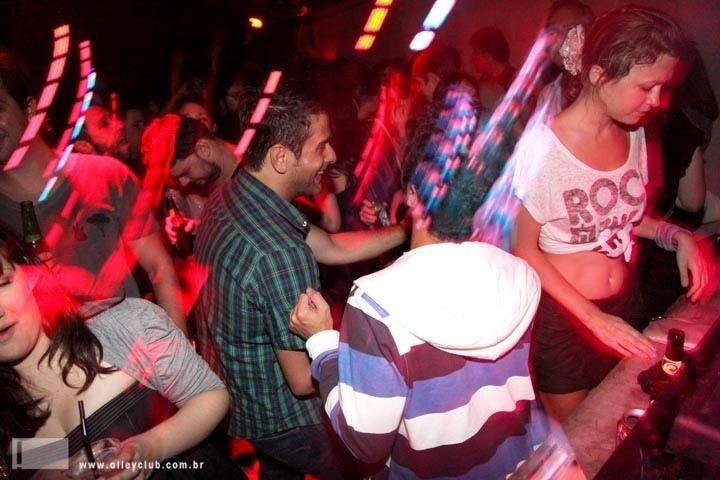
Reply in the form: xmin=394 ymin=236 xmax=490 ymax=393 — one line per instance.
xmin=369 ymin=201 xmax=390 ymax=229
xmin=615 ymin=408 xmax=646 ymax=444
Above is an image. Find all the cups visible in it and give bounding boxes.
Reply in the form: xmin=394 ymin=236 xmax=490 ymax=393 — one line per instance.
xmin=66 ymin=438 xmax=123 ymax=479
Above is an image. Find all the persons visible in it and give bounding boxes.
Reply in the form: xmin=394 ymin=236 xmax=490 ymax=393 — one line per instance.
xmin=0 ymin=1 xmax=720 ymax=479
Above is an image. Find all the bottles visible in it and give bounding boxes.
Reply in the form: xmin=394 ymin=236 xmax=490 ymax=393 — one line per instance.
xmin=18 ymin=201 xmax=40 ymax=257
xmin=642 ymin=327 xmax=692 ymax=461
xmin=167 ymin=195 xmax=194 ymax=255
xmin=365 ymin=185 xmax=390 ymax=227
